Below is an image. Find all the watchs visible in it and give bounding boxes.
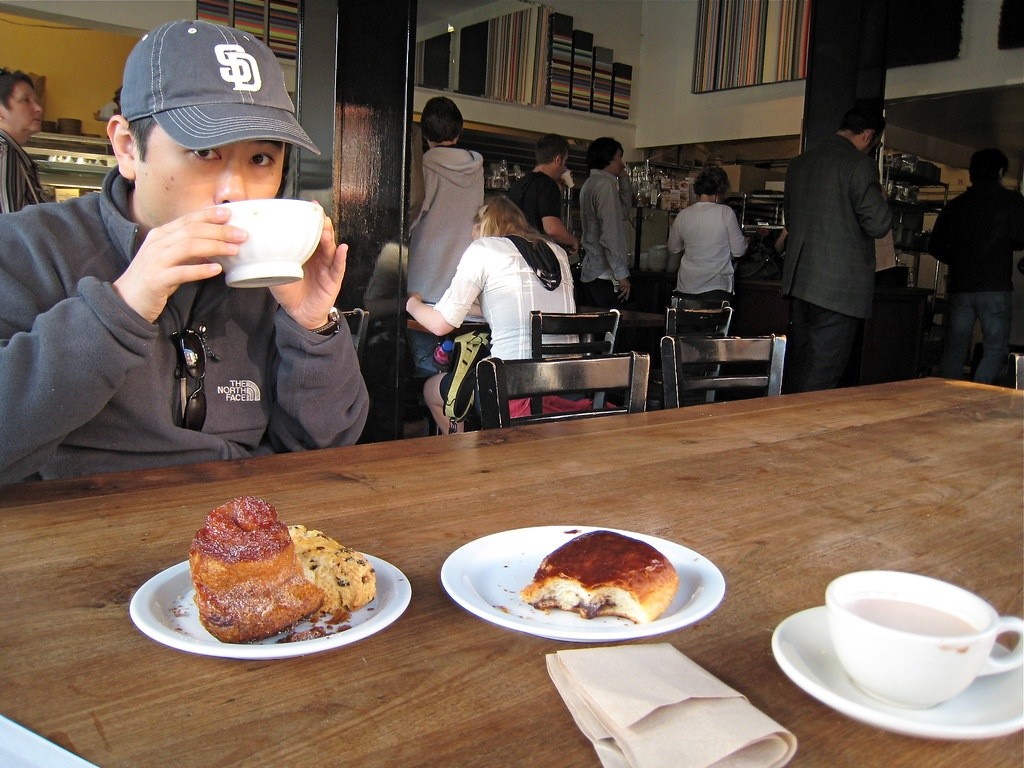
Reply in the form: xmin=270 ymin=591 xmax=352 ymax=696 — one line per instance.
xmin=309 ymin=307 xmax=339 ymax=336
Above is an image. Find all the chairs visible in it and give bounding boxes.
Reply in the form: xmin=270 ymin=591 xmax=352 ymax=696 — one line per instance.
xmin=610 ymin=296 xmax=735 ymax=412
xmin=475 ymin=348 xmax=650 ymax=433
xmin=501 ymin=308 xmax=620 ymax=422
xmin=661 ymin=331 xmax=788 ymax=411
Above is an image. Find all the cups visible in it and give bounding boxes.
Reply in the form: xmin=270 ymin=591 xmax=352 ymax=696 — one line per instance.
xmin=824 ymin=569 xmax=1023 ymax=710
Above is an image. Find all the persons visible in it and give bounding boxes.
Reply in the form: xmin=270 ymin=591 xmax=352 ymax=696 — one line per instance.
xmin=0 ymin=19 xmax=369 ymax=488
xmin=650 ymin=166 xmax=751 ymax=384
xmin=406 ymin=194 xmax=576 ymax=435
xmin=0 ymin=66 xmax=47 ymax=214
xmin=406 ymin=96 xmax=484 ymax=379
xmin=509 ymin=134 xmax=580 ymax=251
xmin=574 ymin=137 xmax=633 ymax=311
xmin=779 ymin=108 xmax=893 ymax=395
xmin=928 ymin=149 xmax=1024 ymax=384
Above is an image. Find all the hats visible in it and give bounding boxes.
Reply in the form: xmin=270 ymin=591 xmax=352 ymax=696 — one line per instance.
xmin=120 ymin=21 xmax=322 ymax=157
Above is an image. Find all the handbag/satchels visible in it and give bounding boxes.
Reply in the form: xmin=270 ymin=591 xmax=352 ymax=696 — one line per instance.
xmin=440 ymin=329 xmax=491 ymax=433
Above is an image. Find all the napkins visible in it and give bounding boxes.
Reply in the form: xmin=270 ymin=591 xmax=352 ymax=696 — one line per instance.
xmin=546 ymin=642 xmax=798 ymax=767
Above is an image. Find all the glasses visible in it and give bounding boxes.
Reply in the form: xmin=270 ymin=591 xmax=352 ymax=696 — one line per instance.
xmin=172 ymin=329 xmax=221 ymax=433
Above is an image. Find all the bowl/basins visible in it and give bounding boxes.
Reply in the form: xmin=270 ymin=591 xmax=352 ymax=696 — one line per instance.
xmin=203 ymin=198 xmax=325 ymax=289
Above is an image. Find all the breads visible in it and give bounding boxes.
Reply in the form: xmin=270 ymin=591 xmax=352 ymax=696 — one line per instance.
xmin=187 ymin=496 xmax=378 ymax=642
xmin=521 ymin=529 xmax=678 ymax=625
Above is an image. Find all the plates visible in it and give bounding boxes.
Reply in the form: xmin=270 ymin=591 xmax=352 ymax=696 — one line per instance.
xmin=128 ymin=552 xmax=413 ymax=660
xmin=770 ymin=605 xmax=1024 ymax=743
xmin=441 ymin=524 xmax=727 ymax=643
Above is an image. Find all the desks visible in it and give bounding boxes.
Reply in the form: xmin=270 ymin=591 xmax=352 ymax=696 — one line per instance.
xmin=404 ymin=307 xmax=665 ymax=435
xmin=0 ymin=379 xmax=1024 ymax=768
